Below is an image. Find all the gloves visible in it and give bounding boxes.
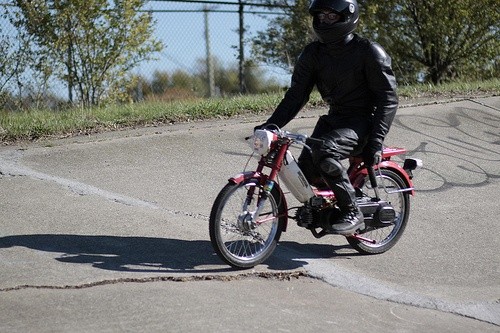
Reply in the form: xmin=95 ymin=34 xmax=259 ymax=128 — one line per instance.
xmin=363 ymin=140 xmax=383 ymax=165
xmin=253 ymin=123 xmax=277 ymax=133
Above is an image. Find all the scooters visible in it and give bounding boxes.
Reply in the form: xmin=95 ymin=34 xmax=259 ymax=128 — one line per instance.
xmin=209 ymin=124 xmax=423 ymax=268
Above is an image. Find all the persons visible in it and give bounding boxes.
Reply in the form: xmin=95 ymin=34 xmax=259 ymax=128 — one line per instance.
xmin=254 ymin=0 xmax=399 ymax=234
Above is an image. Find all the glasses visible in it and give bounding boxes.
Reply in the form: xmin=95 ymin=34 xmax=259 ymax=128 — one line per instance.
xmin=317 ymin=12 xmax=338 ymax=20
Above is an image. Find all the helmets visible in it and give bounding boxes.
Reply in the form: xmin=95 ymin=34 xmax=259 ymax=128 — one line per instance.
xmin=309 ymin=0 xmax=359 ymax=44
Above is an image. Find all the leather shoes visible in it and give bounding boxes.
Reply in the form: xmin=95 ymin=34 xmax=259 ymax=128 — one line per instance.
xmin=331 ymin=206 xmax=365 ymax=234
